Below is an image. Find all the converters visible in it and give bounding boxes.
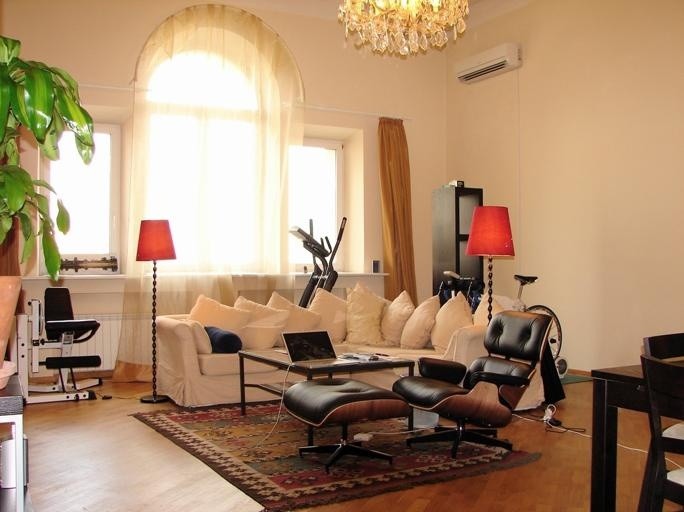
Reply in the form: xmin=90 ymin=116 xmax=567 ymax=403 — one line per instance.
xmin=353 ymin=433 xmax=373 ymax=441
xmin=550 ymin=417 xmax=561 ymax=426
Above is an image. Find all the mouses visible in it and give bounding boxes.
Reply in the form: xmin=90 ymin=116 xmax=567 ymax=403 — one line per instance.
xmin=368 ymin=356 xmax=378 ymax=360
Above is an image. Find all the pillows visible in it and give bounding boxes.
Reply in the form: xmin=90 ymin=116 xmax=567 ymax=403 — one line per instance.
xmin=202 ymin=324 xmax=243 ymax=353
xmin=266 ymin=291 xmax=322 ymax=347
xmin=188 ymin=296 xmax=249 ymax=349
xmin=308 ymin=287 xmax=348 ymax=344
xmin=347 ymin=282 xmax=510 ymax=350
xmin=180 ymin=318 xmax=213 ymax=355
xmin=233 ymin=294 xmax=290 ymax=349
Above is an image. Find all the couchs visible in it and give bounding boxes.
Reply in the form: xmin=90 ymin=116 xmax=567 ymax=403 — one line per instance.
xmin=150 ymin=311 xmax=546 ymax=413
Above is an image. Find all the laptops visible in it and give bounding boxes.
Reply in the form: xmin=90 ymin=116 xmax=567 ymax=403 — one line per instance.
xmin=282 ymin=330 xmax=360 ymax=369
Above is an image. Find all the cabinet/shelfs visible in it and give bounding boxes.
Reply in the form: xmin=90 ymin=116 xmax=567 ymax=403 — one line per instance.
xmin=431 ymin=187 xmax=484 ymax=296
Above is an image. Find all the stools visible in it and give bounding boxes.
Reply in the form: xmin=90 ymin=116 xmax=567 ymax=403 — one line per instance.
xmin=282 ymin=377 xmax=412 ymax=474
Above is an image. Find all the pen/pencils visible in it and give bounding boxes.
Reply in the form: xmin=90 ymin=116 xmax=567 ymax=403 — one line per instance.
xmin=375 ymin=352 xmax=389 ymax=357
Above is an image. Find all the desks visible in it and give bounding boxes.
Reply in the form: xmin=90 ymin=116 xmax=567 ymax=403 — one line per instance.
xmin=591 ymin=361 xmax=683 ymax=512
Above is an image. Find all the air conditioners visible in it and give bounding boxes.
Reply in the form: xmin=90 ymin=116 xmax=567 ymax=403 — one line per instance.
xmin=453 ymin=41 xmax=525 ymax=84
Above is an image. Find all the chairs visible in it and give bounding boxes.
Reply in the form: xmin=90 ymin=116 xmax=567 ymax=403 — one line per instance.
xmin=393 ymin=310 xmax=553 ymax=457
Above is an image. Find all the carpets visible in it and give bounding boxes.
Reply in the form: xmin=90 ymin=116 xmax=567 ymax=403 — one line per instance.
xmin=126 ymin=398 xmax=544 ymax=512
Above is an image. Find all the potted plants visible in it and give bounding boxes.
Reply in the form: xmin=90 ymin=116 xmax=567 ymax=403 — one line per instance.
xmin=0 ymin=34 xmax=97 ymax=375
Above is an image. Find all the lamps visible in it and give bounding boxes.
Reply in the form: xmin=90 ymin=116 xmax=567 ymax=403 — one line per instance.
xmin=137 ymin=218 xmax=178 ymax=403
xmin=338 ymin=0 xmax=468 ymax=57
xmin=464 ymin=206 xmax=516 ymax=325
